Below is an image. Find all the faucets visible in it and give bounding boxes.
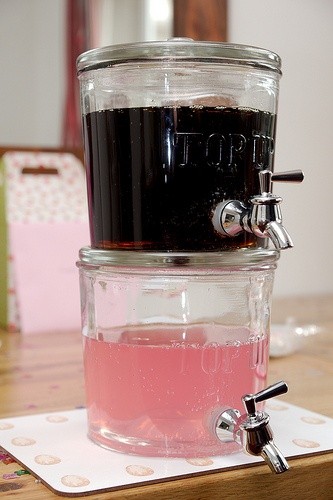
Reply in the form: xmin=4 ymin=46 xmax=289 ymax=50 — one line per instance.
xmin=214 ymin=408 xmax=291 ymax=475
xmin=214 ymin=192 xmax=294 ymax=251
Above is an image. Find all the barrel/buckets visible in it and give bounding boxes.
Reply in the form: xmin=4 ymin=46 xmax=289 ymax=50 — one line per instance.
xmin=74 ymin=247 xmax=290 ymax=474
xmin=77 ymin=37 xmax=304 ymax=251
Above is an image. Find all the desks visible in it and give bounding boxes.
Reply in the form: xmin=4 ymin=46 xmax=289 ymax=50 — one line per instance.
xmin=1 ymin=293 xmax=331 ymax=497
